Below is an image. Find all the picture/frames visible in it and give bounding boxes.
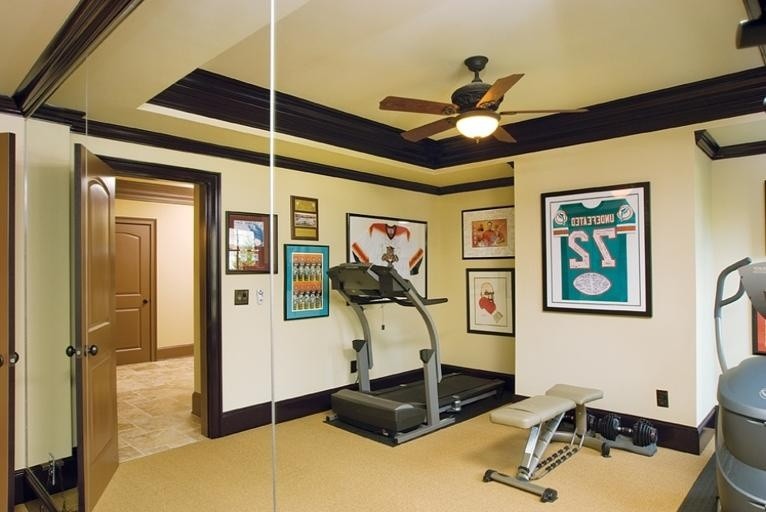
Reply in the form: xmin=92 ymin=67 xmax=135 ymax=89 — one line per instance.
xmin=345 ymin=212 xmax=429 ymax=306
xmin=461 ymin=205 xmax=515 ymax=261
xmin=290 ymin=194 xmax=319 ymax=242
xmin=465 ymin=267 xmax=515 ymax=337
xmin=283 ymin=244 xmax=330 ymax=321
xmin=225 ymin=210 xmax=279 ymax=274
xmin=541 ymin=181 xmax=652 ymax=318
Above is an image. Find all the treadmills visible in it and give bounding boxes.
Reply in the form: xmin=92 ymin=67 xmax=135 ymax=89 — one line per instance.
xmin=327 ymin=262 xmax=505 ymax=446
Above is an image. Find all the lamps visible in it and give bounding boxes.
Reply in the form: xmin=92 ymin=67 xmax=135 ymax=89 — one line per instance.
xmin=454 ymin=110 xmax=500 ymax=143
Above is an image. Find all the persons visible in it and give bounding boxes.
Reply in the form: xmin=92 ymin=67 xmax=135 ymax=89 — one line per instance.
xmin=476 ymin=282 xmax=503 ymax=324
xmin=476 ymin=222 xmax=505 ymax=245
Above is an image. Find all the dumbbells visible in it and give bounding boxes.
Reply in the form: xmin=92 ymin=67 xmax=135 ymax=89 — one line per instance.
xmin=596 ymin=414 xmax=657 ymax=447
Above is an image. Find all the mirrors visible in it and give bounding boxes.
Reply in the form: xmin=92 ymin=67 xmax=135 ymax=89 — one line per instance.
xmin=24 ymin=0 xmax=763 ymax=510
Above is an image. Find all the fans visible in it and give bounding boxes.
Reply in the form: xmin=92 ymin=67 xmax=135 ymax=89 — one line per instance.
xmin=378 ymin=55 xmax=587 ymax=144
